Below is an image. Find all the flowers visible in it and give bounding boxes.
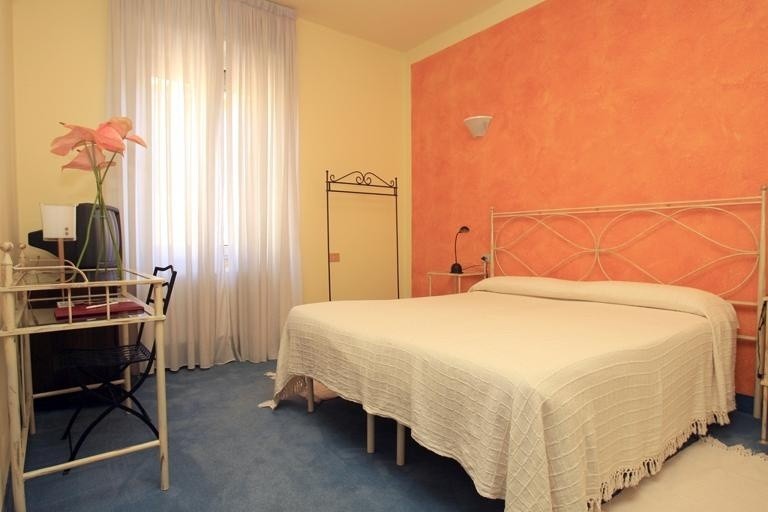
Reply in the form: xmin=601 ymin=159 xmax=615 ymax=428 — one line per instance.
xmin=44 ymin=112 xmax=150 ymax=298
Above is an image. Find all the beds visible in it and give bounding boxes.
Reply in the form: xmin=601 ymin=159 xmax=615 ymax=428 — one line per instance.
xmin=259 ymin=180 xmax=768 ymax=512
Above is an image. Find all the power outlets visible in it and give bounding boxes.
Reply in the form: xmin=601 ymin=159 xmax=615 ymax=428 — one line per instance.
xmin=480 ymin=254 xmax=490 ymax=263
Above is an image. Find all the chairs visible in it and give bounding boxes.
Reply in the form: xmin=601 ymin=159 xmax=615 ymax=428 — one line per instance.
xmin=57 ymin=264 xmax=178 ymax=476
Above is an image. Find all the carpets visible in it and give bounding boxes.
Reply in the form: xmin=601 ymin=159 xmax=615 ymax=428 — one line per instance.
xmin=594 ymin=434 xmax=767 ymax=512
xmin=262 ymin=369 xmax=347 ymax=406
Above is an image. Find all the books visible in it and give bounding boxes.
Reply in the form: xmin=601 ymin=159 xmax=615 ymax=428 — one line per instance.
xmin=54 ymin=301 xmax=144 ymax=320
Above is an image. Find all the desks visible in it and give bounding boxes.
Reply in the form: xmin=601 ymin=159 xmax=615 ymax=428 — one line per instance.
xmin=426 ymin=270 xmax=491 ymax=297
xmin=757 ymin=295 xmax=767 ymax=446
xmin=0 ymin=241 xmax=176 ymax=512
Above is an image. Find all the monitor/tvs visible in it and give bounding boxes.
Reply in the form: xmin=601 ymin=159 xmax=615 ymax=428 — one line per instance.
xmin=27 ymin=202 xmax=123 ymax=269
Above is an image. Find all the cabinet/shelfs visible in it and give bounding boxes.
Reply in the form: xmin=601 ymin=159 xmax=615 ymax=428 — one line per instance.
xmin=24 ymin=302 xmax=125 ymax=412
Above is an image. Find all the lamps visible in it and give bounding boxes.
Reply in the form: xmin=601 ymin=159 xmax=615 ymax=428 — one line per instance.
xmin=448 ymin=225 xmax=471 ymax=274
xmin=463 ymin=113 xmax=493 ymax=139
